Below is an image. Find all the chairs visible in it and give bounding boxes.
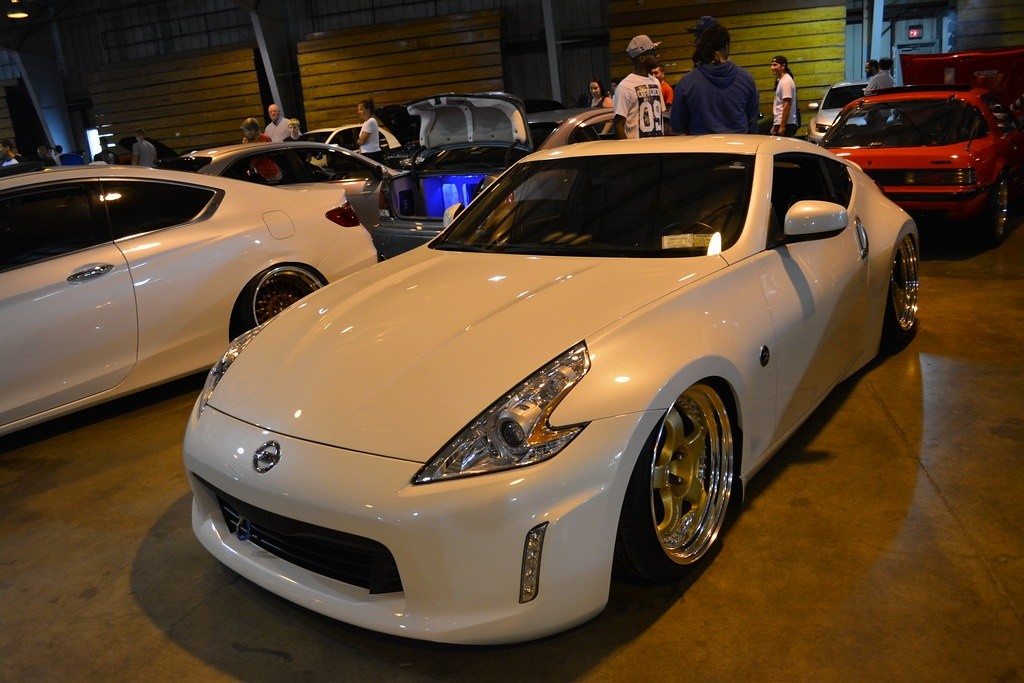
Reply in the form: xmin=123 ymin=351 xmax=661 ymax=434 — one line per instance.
xmin=662 ymin=167 xmax=779 ymax=249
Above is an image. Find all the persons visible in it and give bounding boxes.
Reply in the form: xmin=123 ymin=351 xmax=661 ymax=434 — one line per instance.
xmin=670 ymin=17 xmax=759 ymax=135
xmin=613 ymin=35 xmax=674 ymax=140
xmin=587 ymin=77 xmax=619 ymax=134
xmin=132 ymin=128 xmax=158 ymax=168
xmin=0 ymin=137 xmax=118 ymax=166
xmin=864 ymin=57 xmax=894 ymax=125
xmin=357 ymin=99 xmax=382 ymax=164
xmin=770 ymin=56 xmax=800 ymax=138
xmin=241 ymin=104 xmax=302 ymax=182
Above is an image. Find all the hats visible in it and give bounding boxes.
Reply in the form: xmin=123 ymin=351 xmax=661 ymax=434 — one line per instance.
xmin=627 ymin=35 xmax=663 ymax=58
xmin=772 ymin=55 xmax=789 ymax=69
xmin=687 ymin=15 xmax=716 ymax=33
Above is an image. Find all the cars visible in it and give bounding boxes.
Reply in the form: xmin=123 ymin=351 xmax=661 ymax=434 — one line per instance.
xmin=182 ymin=133 xmax=921 ymax=645
xmin=808 ymin=81 xmax=1024 ymax=246
xmin=0 ymin=165 xmax=382 ymax=442
xmin=175 ymin=92 xmax=619 ymax=261
